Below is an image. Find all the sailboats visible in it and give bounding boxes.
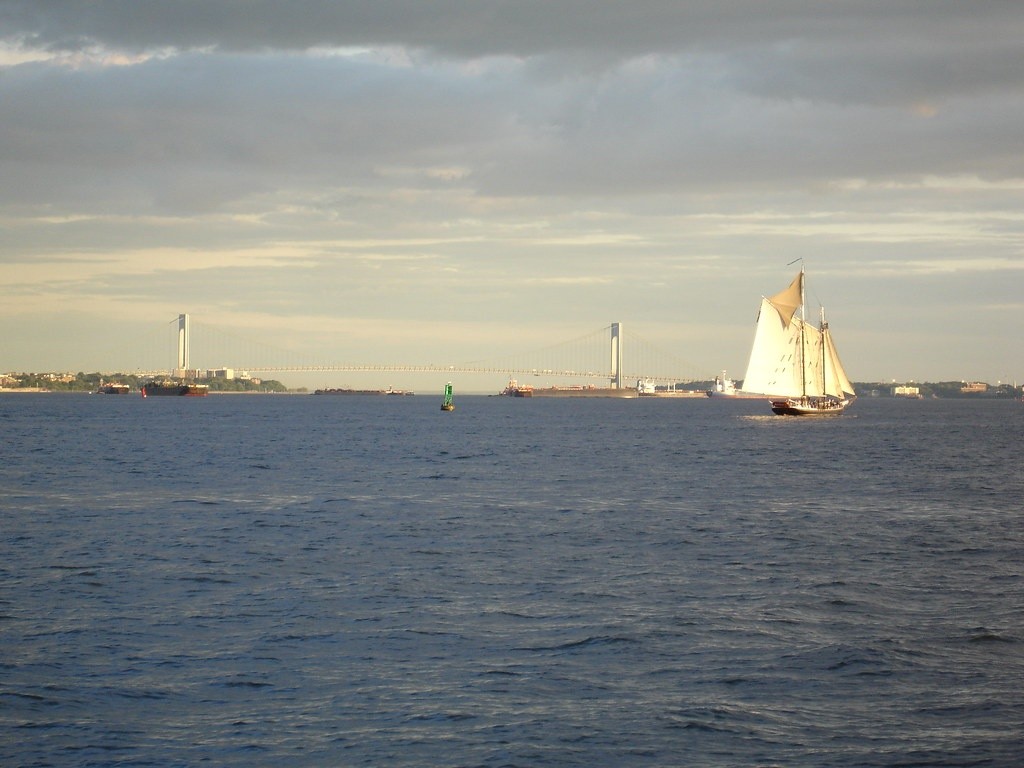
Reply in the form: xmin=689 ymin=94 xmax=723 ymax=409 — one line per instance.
xmin=441 ymin=381 xmax=455 ymax=411
xmin=741 ymin=257 xmax=857 ymax=417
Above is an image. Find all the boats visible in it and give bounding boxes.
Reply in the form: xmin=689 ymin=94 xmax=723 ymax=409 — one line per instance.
xmin=637 ymin=380 xmax=658 ymax=396
xmin=95 ymin=384 xmax=129 ymax=394
xmin=144 ymin=377 xmax=190 ymax=396
xmin=706 ymin=370 xmax=736 ymax=398
xmin=142 ymin=383 xmax=209 ymax=397
